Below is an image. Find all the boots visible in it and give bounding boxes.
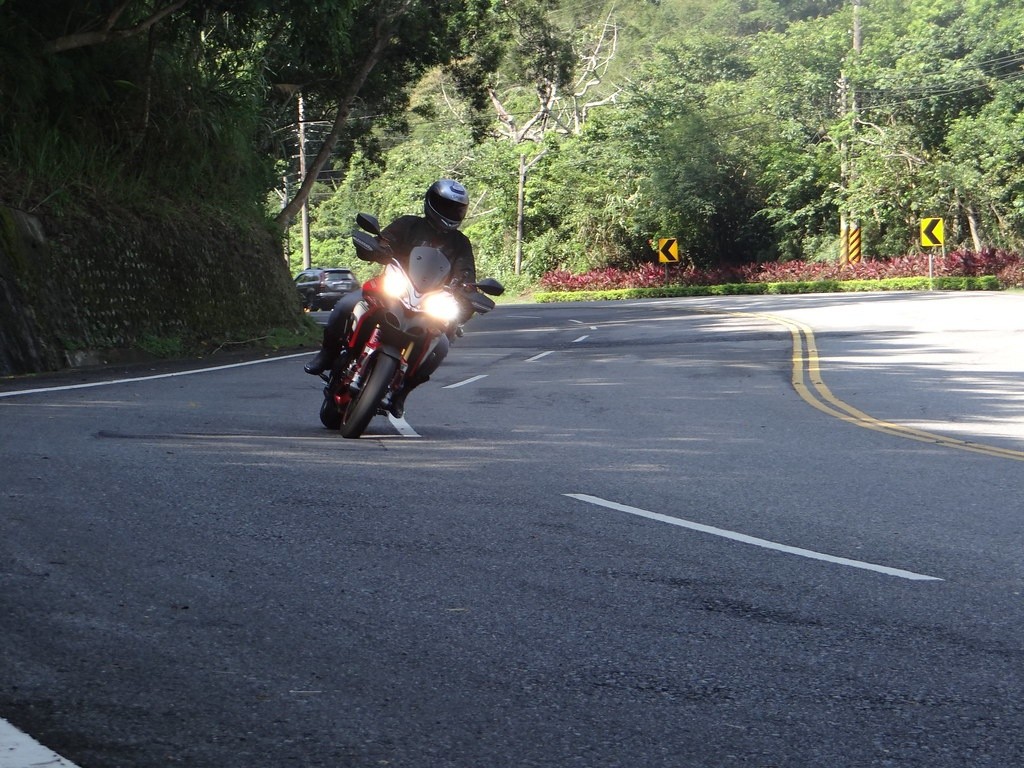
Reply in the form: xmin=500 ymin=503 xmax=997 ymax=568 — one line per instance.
xmin=304 ymin=329 xmax=341 ymax=375
xmin=389 ymin=375 xmax=430 ymax=418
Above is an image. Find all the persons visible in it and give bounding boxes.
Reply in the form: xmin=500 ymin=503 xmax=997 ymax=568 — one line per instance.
xmin=304 ymin=180 xmax=477 ymax=418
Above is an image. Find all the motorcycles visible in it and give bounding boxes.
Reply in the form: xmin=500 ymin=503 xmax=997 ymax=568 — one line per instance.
xmin=318 ymin=213 xmax=505 ymax=439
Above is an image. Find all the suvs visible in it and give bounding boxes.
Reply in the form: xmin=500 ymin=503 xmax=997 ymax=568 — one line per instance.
xmin=293 ymin=267 xmax=360 ymax=312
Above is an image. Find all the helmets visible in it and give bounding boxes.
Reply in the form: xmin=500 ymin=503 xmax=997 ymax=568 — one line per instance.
xmin=424 ymin=181 xmax=469 ymax=234
